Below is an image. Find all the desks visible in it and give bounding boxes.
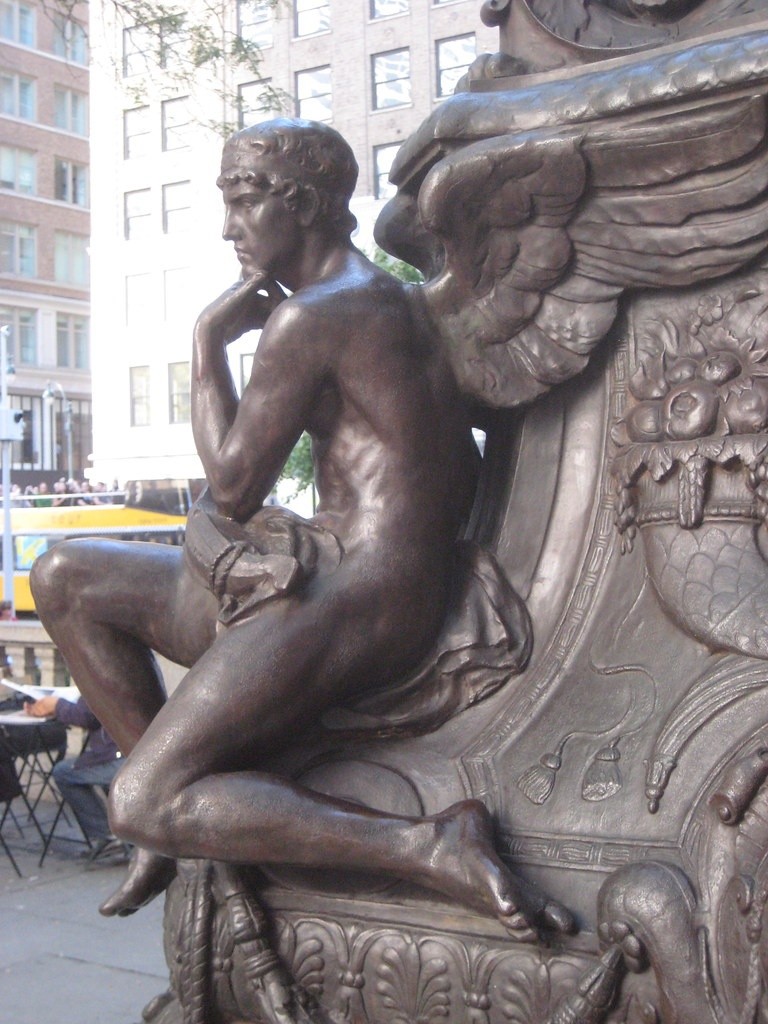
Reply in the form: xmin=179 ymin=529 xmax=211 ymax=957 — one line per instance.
xmin=0 ymin=716 xmax=71 ymax=876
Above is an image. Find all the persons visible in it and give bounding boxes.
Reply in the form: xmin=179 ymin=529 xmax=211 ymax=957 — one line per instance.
xmin=0 ymin=472 xmax=125 ymax=509
xmin=30 ymin=119 xmax=575 ymax=944
xmin=23 ymin=693 xmax=128 ymax=861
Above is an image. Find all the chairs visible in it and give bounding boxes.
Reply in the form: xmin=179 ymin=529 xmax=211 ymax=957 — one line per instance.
xmin=0 ymin=705 xmax=142 ymax=876
